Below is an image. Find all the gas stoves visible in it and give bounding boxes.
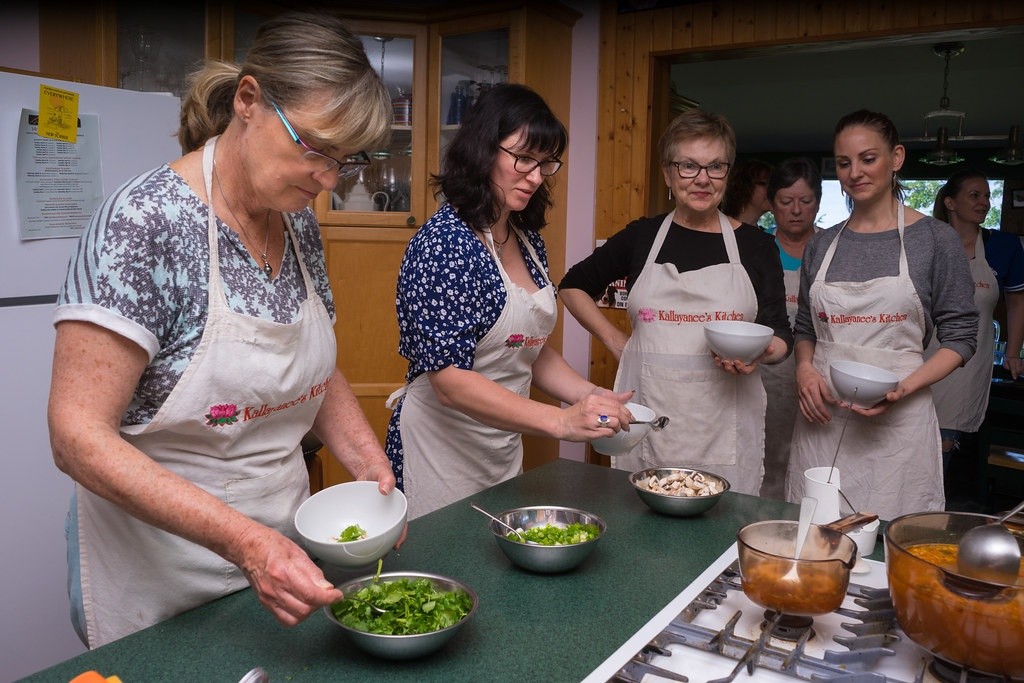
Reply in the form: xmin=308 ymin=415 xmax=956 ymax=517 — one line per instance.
xmin=570 ymin=533 xmax=1024 ymax=683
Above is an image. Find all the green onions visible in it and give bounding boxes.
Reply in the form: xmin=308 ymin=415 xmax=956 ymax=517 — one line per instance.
xmin=505 ymin=522 xmax=599 ymax=545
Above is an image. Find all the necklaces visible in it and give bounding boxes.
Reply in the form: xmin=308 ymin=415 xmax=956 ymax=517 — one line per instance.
xmin=885 ymin=209 xmax=898 ymax=230
xmin=493 ymin=219 xmax=509 ymax=260
xmin=214 ymin=162 xmax=273 ymax=275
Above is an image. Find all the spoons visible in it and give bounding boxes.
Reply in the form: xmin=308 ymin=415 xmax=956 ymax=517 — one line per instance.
xmin=344 ymin=595 xmax=390 ymax=613
xmin=629 ymin=416 xmax=671 ymax=431
xmin=470 ymin=501 xmax=539 ymax=545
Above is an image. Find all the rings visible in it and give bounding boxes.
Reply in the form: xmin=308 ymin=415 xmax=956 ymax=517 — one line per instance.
xmin=598 ymin=415 xmax=610 ymax=428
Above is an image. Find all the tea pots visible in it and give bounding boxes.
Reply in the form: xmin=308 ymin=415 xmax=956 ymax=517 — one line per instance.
xmin=329 ymin=179 xmax=390 ymax=213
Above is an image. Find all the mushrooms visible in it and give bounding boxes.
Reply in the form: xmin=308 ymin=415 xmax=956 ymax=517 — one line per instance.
xmin=636 ymin=471 xmax=719 ymax=497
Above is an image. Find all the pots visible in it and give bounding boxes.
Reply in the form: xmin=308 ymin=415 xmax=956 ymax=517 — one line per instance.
xmin=736 ymin=510 xmax=878 ymax=616
xmin=881 ymin=510 xmax=1024 ymax=681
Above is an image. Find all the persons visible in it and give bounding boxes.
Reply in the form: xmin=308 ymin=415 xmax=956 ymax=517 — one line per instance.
xmin=49 ymin=18 xmax=408 ymax=645
xmin=385 ymin=84 xmax=635 ymax=520
xmin=760 ymin=160 xmax=825 ymax=499
xmin=921 ymin=171 xmax=1024 ymax=474
xmin=557 ymin=109 xmax=794 ymax=494
xmin=785 ymin=110 xmax=980 ymax=522
xmin=724 ymin=159 xmax=773 ymax=226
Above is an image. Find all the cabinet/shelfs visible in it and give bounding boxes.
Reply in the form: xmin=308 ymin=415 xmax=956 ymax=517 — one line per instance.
xmin=39 ymin=0 xmax=583 ymax=492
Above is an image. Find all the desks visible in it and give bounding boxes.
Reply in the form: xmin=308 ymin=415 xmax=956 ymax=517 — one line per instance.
xmin=11 ymin=454 xmax=936 ymax=682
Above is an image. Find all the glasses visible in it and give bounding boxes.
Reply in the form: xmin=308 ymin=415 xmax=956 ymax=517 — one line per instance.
xmin=272 ymin=101 xmax=372 ymax=178
xmin=667 ymin=158 xmax=730 ymax=179
xmin=500 ymin=146 xmax=563 ymax=176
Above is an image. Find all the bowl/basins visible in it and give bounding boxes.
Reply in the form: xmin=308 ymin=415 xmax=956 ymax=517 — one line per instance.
xmin=829 ymin=360 xmax=903 ymax=409
xmin=488 ymin=505 xmax=608 ymax=573
xmin=294 ymin=480 xmax=408 ymax=567
xmin=627 ymin=467 xmax=730 ymax=517
xmin=591 ymin=401 xmax=656 ymax=455
xmin=322 ymin=571 xmax=478 ymax=654
xmin=703 ymin=319 xmax=775 ymax=365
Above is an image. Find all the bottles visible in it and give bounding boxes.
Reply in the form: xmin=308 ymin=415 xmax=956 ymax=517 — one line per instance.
xmin=336 ymin=145 xmax=413 ymax=213
xmin=445 ymin=65 xmax=509 ymax=125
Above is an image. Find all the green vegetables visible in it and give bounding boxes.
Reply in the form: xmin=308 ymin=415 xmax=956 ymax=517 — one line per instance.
xmin=332 ymin=558 xmax=470 ymax=636
xmin=338 ymin=524 xmax=367 ymax=543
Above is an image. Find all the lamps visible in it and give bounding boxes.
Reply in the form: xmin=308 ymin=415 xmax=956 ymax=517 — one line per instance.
xmin=897 ymin=40 xmax=1024 ymax=165
